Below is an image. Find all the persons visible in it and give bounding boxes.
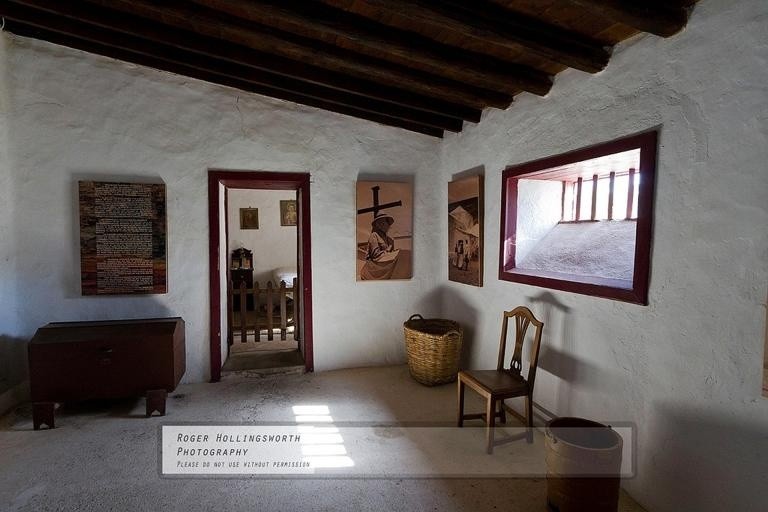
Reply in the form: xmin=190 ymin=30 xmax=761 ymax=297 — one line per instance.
xmin=285 ymin=202 xmax=297 ymax=225
xmin=463 ymin=240 xmax=470 ymax=271
xmin=360 ymin=210 xmax=400 ymax=281
xmin=456 ymin=240 xmax=463 ymax=270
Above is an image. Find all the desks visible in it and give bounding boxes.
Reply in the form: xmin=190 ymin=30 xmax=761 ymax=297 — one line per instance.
xmin=27 ymin=317 xmax=187 ymax=434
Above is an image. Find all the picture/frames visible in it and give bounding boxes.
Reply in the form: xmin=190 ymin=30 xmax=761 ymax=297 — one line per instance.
xmin=279 ymin=199 xmax=297 ymax=227
xmin=446 ymin=174 xmax=485 ymax=289
xmin=240 ymin=207 xmax=260 ymax=231
xmin=355 ymin=180 xmax=415 ymax=284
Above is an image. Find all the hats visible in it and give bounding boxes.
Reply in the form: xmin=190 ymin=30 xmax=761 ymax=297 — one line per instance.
xmin=371 ymin=208 xmax=395 ymax=227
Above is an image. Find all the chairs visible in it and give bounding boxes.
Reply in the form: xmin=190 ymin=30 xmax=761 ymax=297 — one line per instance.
xmin=455 ymin=307 xmax=544 ymax=456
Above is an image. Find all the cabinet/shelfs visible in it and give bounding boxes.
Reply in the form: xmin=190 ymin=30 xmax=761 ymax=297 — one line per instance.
xmin=230 ymin=248 xmax=255 ymax=314
